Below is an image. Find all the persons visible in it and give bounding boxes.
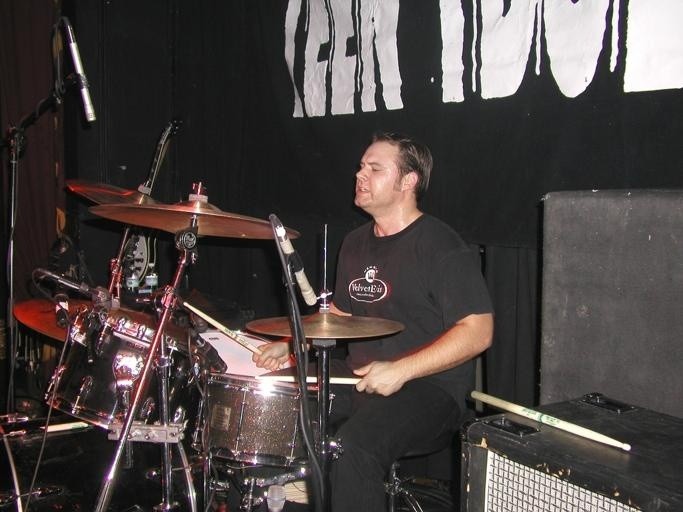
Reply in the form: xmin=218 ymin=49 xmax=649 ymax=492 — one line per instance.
xmin=253 ymin=128 xmax=495 ymax=512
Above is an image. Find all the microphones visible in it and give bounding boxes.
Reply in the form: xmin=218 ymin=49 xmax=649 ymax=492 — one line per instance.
xmin=191 ymin=328 xmax=227 ymax=373
xmin=270 ymin=214 xmax=317 ymax=306
xmin=54 ymin=285 xmax=69 ymax=328
xmin=38 ymin=267 xmax=107 ymax=303
xmin=268 ymin=484 xmax=286 ymax=512
xmin=63 ymin=17 xmax=97 ymax=122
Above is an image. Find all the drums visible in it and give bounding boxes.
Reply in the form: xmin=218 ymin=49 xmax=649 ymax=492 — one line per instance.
xmin=190 ymin=371 xmax=335 ymax=470
xmin=44 ymin=304 xmax=201 ymax=442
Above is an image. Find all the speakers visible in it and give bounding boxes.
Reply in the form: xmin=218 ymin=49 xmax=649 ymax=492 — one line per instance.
xmin=459 ymin=391 xmax=683 ymax=512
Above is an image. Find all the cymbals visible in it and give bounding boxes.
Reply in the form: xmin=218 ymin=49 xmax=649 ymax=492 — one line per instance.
xmin=88 ymin=200 xmax=301 ymax=241
xmin=246 ymin=312 xmax=404 ymax=340
xmin=65 ymin=178 xmax=163 ymax=206
xmin=13 ymin=298 xmax=195 ymax=353
